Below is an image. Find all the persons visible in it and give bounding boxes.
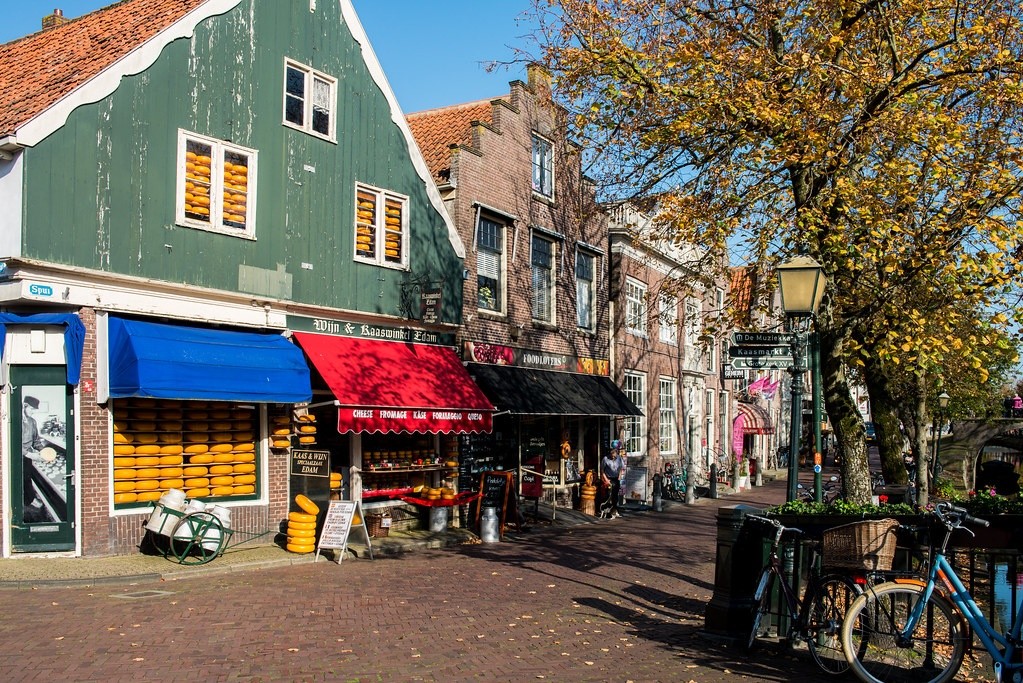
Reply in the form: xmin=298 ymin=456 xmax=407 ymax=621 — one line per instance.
xmin=20 ymin=396 xmax=43 ymax=506
xmin=600 ymin=446 xmax=623 ymax=519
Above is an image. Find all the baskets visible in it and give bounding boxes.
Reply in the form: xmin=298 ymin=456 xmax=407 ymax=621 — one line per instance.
xmin=821 ymin=518 xmax=901 ymax=572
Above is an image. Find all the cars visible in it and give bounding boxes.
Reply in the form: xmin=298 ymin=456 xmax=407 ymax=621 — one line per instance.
xmin=864 ymin=421 xmax=876 ymax=444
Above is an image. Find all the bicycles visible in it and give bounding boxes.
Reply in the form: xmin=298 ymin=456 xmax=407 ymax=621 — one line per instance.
xmin=870 ymin=451 xmax=933 ymax=494
xmin=668 ymin=462 xmax=699 ymax=503
xmin=777 ymin=445 xmax=789 ymax=468
xmin=841 ymin=501 xmax=1023 ymax=683
xmin=744 ymin=512 xmax=900 ymax=678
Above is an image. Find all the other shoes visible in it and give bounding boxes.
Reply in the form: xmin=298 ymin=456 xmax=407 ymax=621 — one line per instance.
xmin=612 ymin=510 xmax=622 ymax=518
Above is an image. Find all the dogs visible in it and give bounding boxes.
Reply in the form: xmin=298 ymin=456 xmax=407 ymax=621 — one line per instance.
xmin=599 ymin=497 xmax=623 ymax=520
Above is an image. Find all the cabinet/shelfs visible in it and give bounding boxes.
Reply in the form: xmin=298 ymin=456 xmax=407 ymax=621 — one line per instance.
xmin=185 ymin=159 xmax=245 ymax=225
xmin=269 ymin=420 xmax=318 ymax=449
xmin=357 ymin=195 xmax=403 ymax=259
xmin=361 ymin=463 xmax=454 ymax=497
xmin=113 ymin=404 xmax=257 ymax=505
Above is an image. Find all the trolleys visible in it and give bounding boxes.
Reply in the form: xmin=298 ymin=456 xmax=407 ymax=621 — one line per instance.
xmin=147 ymin=500 xmax=271 ymax=565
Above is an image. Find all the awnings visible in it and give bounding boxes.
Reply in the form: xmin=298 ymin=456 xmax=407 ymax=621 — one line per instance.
xmin=736 ymin=402 xmax=775 ymax=433
xmin=464 ymin=362 xmax=645 ymax=416
xmin=108 ymin=315 xmax=310 ymax=402
xmin=291 ymin=330 xmax=495 ymax=434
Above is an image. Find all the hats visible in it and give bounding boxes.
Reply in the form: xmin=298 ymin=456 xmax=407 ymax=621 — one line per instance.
xmin=24 ymin=396 xmax=39 ymax=409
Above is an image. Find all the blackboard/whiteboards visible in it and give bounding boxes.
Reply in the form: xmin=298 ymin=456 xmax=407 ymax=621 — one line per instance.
xmin=474 ymin=471 xmax=522 ymax=537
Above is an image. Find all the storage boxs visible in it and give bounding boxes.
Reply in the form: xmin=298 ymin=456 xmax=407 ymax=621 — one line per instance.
xmin=821 ymin=517 xmax=900 ymax=572
xmin=363 ymin=514 xmax=390 ymax=538
xmin=480 ymin=507 xmax=500 ymax=543
xmin=430 ymin=506 xmax=447 ymax=536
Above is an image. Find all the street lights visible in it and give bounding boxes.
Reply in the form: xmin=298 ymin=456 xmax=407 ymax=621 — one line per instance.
xmin=777 ymin=245 xmax=828 ymax=502
xmin=931 ymin=390 xmax=951 ymax=495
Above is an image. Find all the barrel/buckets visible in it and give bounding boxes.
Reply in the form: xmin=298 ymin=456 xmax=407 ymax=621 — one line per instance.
xmin=172 ymin=498 xmax=209 ymax=543
xmin=144 ymin=487 xmax=189 ymax=538
xmin=196 ymin=504 xmax=231 ymax=553
xmin=480 ymin=507 xmax=499 ymax=542
xmin=429 ymin=506 xmax=448 ymax=533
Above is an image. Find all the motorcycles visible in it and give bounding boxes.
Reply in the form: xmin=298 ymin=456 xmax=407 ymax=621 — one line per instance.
xmin=798 ymin=475 xmax=838 ymax=503
